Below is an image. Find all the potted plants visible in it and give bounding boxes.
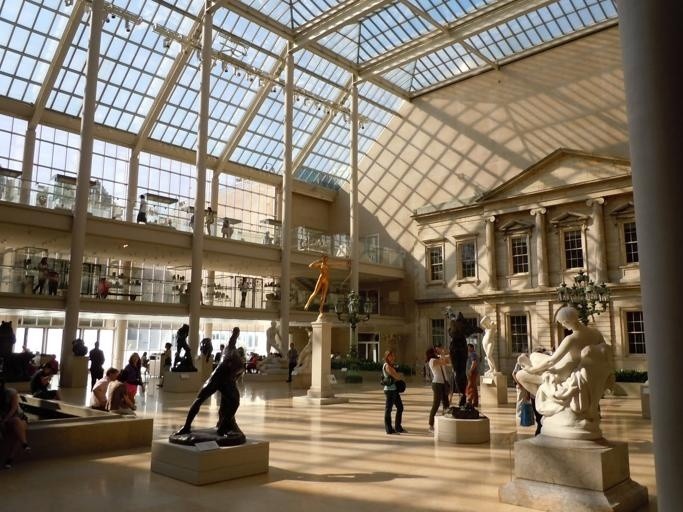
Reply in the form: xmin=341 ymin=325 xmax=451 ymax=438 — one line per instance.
xmin=331 ymin=359 xmax=417 ymax=383
xmin=614 ymin=368 xmax=648 ymax=395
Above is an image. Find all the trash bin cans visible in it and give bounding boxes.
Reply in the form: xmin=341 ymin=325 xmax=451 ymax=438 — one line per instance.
xmin=149 ymin=352 xmax=165 ymax=379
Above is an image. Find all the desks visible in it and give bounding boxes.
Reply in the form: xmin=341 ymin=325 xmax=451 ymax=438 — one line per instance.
xmin=244 ymin=362 xmax=254 ymax=374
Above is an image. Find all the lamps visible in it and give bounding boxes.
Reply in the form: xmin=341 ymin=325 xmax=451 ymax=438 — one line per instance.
xmin=336 ymin=289 xmax=373 ymax=383
xmin=81 ymin=0 xmax=142 ymax=31
xmin=555 ymin=270 xmax=611 ymax=327
xmin=152 ymin=23 xmax=369 ymax=130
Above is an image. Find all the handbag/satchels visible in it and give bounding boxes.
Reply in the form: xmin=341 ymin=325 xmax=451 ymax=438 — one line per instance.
xmin=380 ymin=375 xmax=393 ymax=386
xmin=521 ymin=403 xmax=534 ymax=426
xmin=444 ymin=382 xmax=450 ymax=394
xmin=450 ymin=406 xmax=479 ymax=418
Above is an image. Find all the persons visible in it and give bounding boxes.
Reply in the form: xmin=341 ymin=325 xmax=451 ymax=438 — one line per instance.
xmin=97 ymin=277 xmax=110 ymax=299
xmin=477 ymin=315 xmax=501 ymax=379
xmin=160 ymin=343 xmax=172 ymax=388
xmin=174 ymin=324 xmax=191 ymax=368
xmin=441 ymin=304 xmax=472 ymax=411
xmin=133 ymin=194 xmax=148 ymax=225
xmin=0 ymin=386 xmax=31 ymax=467
xmin=287 ymin=343 xmax=298 ymax=383
xmin=527 ymin=346 xmax=543 ymax=438
xmin=267 ymin=321 xmax=283 ymax=356
xmin=220 ymin=217 xmax=230 ymax=239
xmin=31 ymin=361 xmax=59 ymax=400
xmin=426 ymin=345 xmax=453 ymax=431
xmin=302 ymin=252 xmax=342 ymax=314
xmin=177 ymin=326 xmax=247 ymax=436
xmin=382 ymin=351 xmax=406 ymax=433
xmin=236 ymin=275 xmax=249 ymax=307
xmin=89 ymin=342 xmax=149 ymax=415
xmin=514 ymin=304 xmax=613 ymax=440
xmin=46 ymin=268 xmax=59 ymax=296
xmin=203 ymin=205 xmax=217 ymax=236
xmin=247 ymin=352 xmax=258 ymax=373
xmin=31 ymin=256 xmax=48 ymax=295
